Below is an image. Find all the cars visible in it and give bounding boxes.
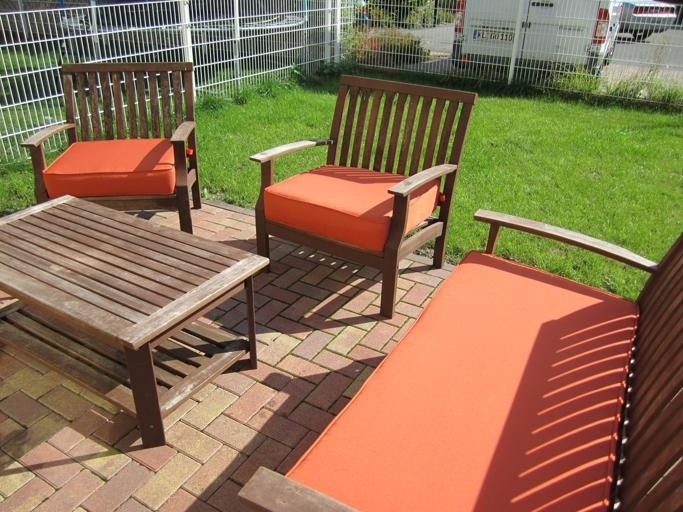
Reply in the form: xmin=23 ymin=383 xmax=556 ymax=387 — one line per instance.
xmin=619 ymin=0 xmax=677 ymax=38
xmin=355 ymin=0 xmax=372 ymax=35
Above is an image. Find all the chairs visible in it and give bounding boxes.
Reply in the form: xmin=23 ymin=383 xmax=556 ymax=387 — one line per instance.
xmin=250 ymin=75 xmax=476 ymax=319
xmin=20 ymin=62 xmax=201 ymax=235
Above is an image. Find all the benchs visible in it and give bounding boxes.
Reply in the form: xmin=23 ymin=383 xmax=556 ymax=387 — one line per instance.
xmin=236 ymin=208 xmax=683 ymax=512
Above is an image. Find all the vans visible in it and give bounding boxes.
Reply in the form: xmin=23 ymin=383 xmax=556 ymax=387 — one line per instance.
xmin=452 ymin=1 xmax=623 ymax=81
